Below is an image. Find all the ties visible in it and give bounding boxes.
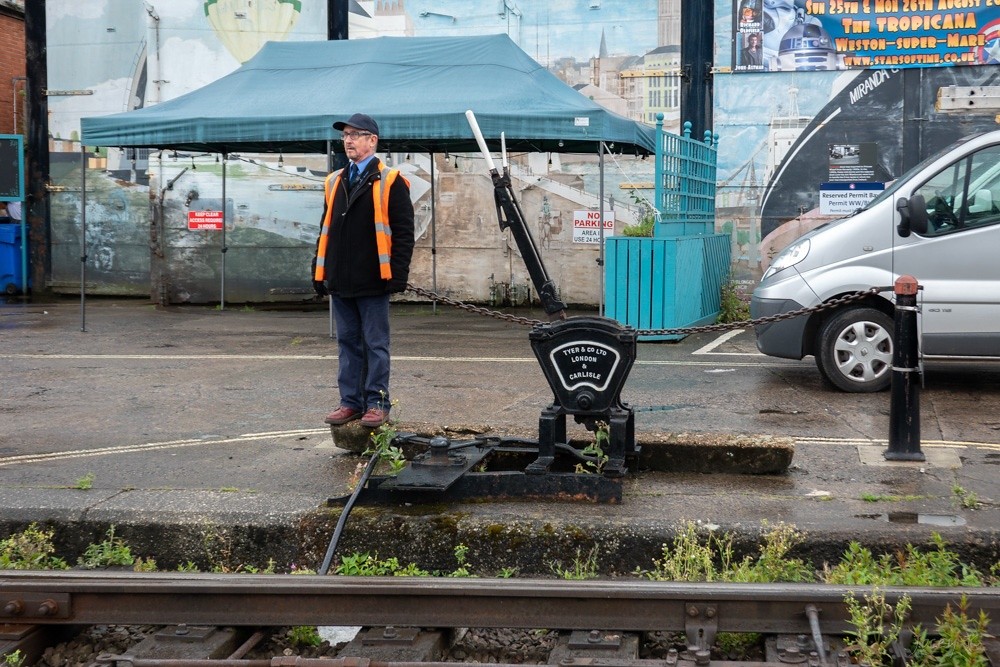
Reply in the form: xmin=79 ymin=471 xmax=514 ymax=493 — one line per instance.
xmin=349 ymin=164 xmax=358 ymax=185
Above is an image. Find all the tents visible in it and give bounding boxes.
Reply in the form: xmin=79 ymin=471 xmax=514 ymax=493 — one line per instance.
xmin=78 ymin=32 xmax=656 ymax=333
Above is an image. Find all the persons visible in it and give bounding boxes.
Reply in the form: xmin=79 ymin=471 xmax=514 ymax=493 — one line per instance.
xmin=312 ymin=113 xmax=417 ymax=427
xmin=739 ymin=0 xmax=762 ymax=29
xmin=741 ymin=33 xmax=762 ymax=65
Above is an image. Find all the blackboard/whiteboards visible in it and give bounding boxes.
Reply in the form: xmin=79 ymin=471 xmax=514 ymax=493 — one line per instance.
xmin=0 ymin=134 xmax=24 ymax=201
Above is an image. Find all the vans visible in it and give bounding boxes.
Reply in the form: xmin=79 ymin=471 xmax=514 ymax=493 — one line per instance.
xmin=750 ymin=125 xmax=1000 ymax=393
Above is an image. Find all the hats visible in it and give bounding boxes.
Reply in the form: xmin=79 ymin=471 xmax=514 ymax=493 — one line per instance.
xmin=333 ymin=113 xmax=380 ymax=137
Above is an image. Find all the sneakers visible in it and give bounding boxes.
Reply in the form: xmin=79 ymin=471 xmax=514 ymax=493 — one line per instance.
xmin=324 ymin=404 xmax=356 ymax=424
xmin=361 ymin=408 xmax=389 ymax=426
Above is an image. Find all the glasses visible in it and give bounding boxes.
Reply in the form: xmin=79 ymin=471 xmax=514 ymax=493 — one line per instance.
xmin=339 ymin=131 xmax=371 ymax=140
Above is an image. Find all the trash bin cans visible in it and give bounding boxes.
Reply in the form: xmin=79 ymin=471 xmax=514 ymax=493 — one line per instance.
xmin=0 ymin=223 xmax=27 ymax=292
